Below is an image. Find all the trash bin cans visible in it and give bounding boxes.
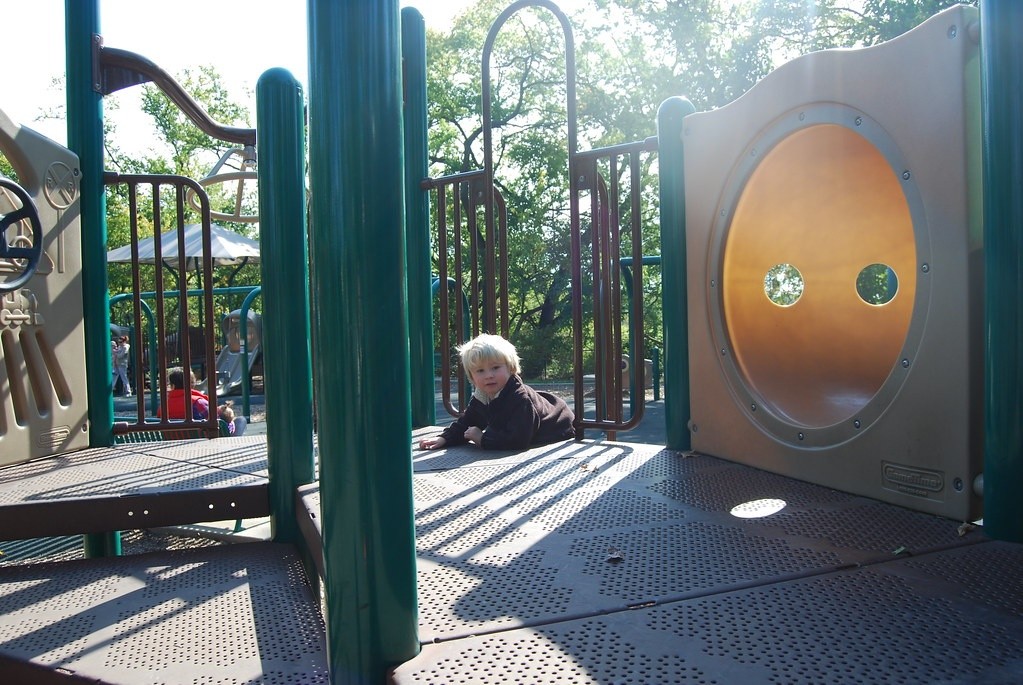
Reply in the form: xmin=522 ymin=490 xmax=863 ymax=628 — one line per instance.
xmin=643 ymin=358 xmax=654 ymax=389
xmin=621 ymin=354 xmax=629 ymax=389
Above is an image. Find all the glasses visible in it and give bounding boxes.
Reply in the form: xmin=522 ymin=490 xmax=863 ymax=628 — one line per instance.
xmin=118 ymin=341 xmax=124 ymax=344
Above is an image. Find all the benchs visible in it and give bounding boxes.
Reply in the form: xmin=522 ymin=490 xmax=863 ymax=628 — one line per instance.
xmin=114 ymin=416 xmax=231 ymax=444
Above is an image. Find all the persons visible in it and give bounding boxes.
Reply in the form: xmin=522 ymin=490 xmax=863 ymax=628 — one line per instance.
xmin=111 ymin=336 xmax=132 ymax=398
xmin=420 ymin=334 xmax=574 ymax=449
xmin=217 ymin=401 xmax=234 ymax=421
xmin=157 ymin=367 xmax=247 ymax=436
xmin=111 ymin=341 xmax=120 ymax=374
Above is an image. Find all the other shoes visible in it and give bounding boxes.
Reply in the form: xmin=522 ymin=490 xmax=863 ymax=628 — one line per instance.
xmin=125 ymin=394 xmax=132 ymax=398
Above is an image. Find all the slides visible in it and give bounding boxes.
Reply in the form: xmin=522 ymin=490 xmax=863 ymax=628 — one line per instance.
xmin=193 ymin=344 xmax=260 ymax=397
